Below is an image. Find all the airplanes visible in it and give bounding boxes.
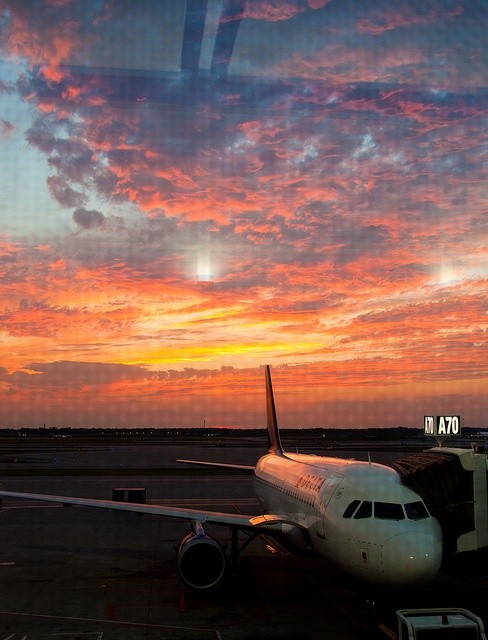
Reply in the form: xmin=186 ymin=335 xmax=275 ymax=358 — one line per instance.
xmin=0 ymin=364 xmax=488 ymax=588
